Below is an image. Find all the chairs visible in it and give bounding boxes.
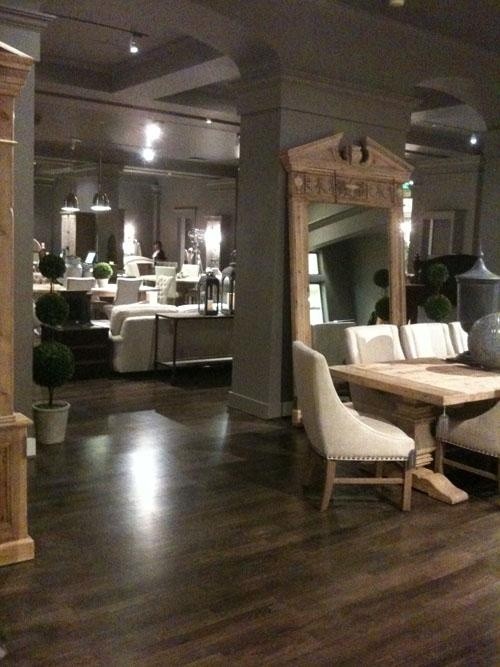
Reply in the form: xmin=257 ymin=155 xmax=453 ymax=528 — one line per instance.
xmin=31 ymin=246 xmax=202 ymax=322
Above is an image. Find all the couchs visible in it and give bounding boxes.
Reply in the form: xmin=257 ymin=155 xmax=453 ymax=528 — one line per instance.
xmin=96 ymin=301 xmax=235 ymax=372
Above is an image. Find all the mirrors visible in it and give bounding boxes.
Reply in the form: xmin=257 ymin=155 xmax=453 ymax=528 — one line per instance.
xmin=283 ymin=129 xmax=416 ymax=426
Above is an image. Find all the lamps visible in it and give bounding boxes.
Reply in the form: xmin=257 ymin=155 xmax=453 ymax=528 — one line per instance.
xmin=61 ymin=152 xmax=112 ymax=212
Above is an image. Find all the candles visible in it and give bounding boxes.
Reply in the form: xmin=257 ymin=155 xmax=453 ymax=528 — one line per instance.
xmin=226 ymin=292 xmax=235 ymax=304
xmin=206 ymin=299 xmax=213 ymax=310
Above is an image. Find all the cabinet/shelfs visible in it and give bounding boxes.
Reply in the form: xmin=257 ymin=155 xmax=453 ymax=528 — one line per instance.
xmin=152 ymin=312 xmax=235 ymax=385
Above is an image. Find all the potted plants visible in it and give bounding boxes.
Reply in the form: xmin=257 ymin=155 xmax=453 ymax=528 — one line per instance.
xmin=31 ymin=252 xmax=79 ymax=445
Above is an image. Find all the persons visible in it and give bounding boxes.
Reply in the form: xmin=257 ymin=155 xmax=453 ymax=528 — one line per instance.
xmin=151 ymin=239 xmax=166 ymax=260
